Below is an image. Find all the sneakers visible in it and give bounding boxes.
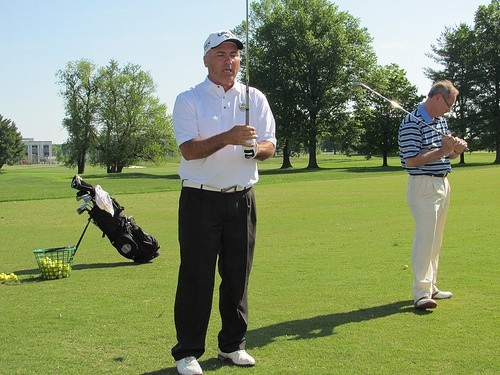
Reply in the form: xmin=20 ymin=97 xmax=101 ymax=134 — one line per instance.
xmin=174 ymin=355 xmax=203 ymax=375
xmin=217 ymin=337 xmax=256 ymax=365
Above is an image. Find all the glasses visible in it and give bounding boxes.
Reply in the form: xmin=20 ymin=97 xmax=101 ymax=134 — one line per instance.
xmin=442 ymin=94 xmax=455 ymax=111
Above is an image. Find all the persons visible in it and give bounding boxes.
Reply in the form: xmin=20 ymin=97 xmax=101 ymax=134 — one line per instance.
xmin=398 ymin=82 xmax=468 ymax=308
xmin=172 ymin=30 xmax=277 ymax=374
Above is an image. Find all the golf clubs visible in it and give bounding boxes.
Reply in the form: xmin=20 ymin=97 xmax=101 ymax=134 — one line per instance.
xmin=245 ymin=0 xmax=250 ymax=125
xmin=71 ymin=174 xmax=95 ymax=215
xmin=352 ymin=82 xmax=470 ymax=153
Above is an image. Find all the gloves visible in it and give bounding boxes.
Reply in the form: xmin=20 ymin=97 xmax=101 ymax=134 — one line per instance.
xmin=243 ymin=138 xmax=259 ymax=159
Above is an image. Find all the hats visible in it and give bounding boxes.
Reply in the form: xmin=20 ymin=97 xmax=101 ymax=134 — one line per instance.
xmin=204 ymin=30 xmax=244 ymax=57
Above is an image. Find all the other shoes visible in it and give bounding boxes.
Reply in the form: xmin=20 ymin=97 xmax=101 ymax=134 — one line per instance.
xmin=433 ymin=291 xmax=453 ymax=299
xmin=415 ymin=297 xmax=437 ymax=309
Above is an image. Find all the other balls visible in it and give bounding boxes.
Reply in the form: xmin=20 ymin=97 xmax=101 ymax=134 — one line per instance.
xmin=0 ymin=257 xmax=72 ymax=284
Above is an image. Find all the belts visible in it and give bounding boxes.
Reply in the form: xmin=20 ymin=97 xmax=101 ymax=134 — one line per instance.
xmin=410 ymin=173 xmax=447 ymax=177
xmin=182 ymin=179 xmax=253 ymax=194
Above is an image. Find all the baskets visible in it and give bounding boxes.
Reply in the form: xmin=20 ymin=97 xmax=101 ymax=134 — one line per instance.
xmin=33 ymin=245 xmax=76 ymax=280
